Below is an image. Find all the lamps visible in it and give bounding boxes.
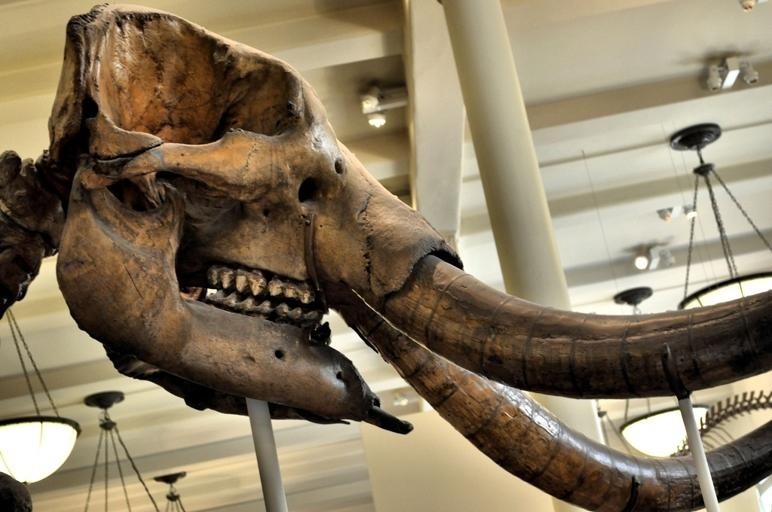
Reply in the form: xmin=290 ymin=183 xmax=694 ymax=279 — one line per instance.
xmin=707 ymin=55 xmax=761 ymax=93
xmin=633 ymin=244 xmax=676 ymax=270
xmin=669 ymin=124 xmax=772 ymax=311
xmin=1 ymin=308 xmax=82 ymax=485
xmin=657 ymin=206 xmax=696 ymax=221
xmin=361 ymin=81 xmax=408 ymax=127
xmin=613 ymin=287 xmax=708 ymax=457
